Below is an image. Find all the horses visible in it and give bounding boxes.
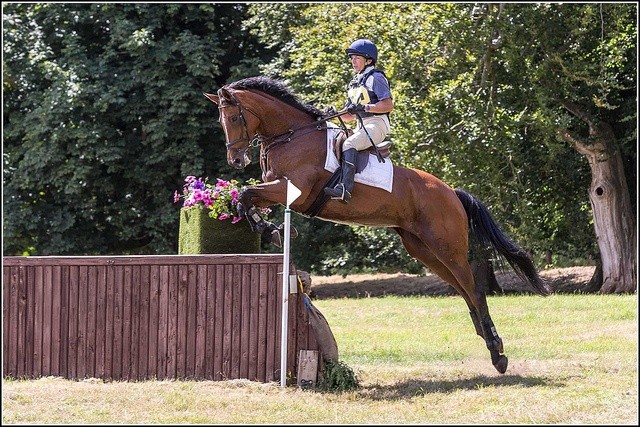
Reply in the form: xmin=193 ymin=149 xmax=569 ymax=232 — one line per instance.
xmin=201 ymin=74 xmax=551 ymax=374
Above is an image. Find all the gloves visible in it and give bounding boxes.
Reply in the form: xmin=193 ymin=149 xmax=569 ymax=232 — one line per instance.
xmin=348 ymin=103 xmax=365 ymax=114
xmin=325 ymin=105 xmax=338 ymax=116
xmin=346 ymin=97 xmax=351 ymax=107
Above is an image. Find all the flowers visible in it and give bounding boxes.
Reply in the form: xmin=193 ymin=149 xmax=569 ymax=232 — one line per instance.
xmin=174 ymin=175 xmax=272 ymax=225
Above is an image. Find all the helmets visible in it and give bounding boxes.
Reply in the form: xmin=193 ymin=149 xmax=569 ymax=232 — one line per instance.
xmin=345 ymin=39 xmax=378 ymax=62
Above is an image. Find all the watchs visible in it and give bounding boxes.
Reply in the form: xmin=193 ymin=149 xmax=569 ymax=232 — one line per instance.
xmin=364 ymin=103 xmax=371 ymax=112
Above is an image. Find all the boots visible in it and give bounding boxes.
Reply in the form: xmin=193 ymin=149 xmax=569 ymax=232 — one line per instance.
xmin=324 ymin=148 xmax=359 ymax=204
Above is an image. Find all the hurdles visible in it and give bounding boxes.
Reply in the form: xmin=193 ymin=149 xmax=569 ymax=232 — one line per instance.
xmin=281 ymin=208 xmax=291 ymax=386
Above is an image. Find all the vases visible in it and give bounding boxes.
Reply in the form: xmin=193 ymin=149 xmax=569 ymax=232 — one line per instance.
xmin=178 ymin=207 xmax=261 ymax=254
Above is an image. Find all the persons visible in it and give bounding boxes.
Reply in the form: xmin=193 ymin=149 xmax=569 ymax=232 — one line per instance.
xmin=322 ymin=38 xmax=395 ymax=204
